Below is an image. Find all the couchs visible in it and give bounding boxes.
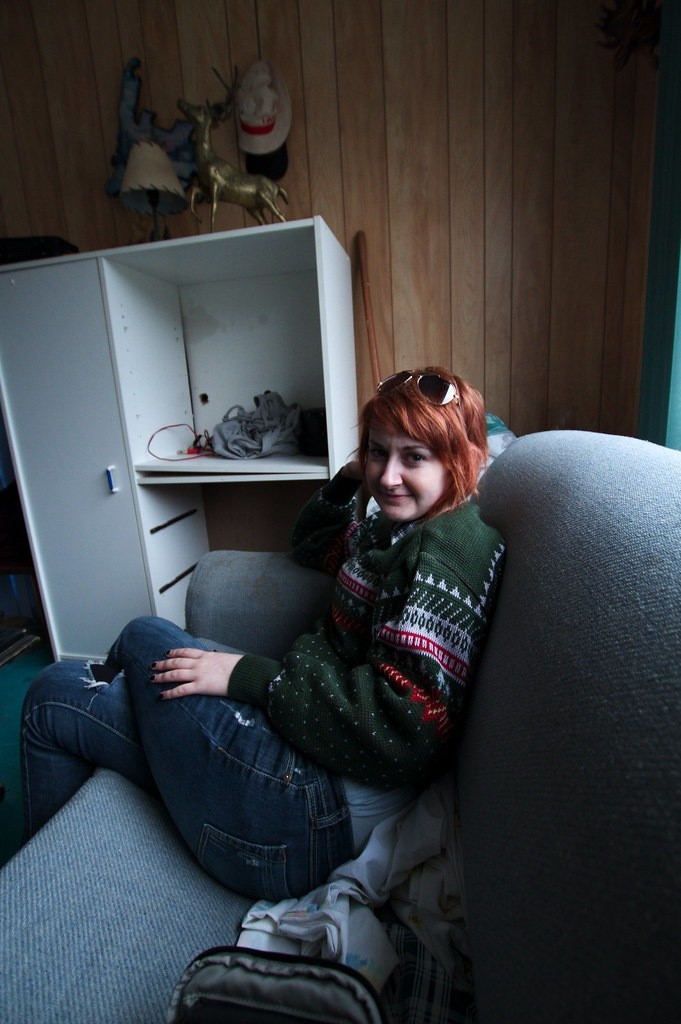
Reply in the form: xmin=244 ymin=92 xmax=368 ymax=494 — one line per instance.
xmin=0 ymin=417 xmax=681 ymax=1024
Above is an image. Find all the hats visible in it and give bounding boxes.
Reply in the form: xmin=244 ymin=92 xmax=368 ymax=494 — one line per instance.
xmin=234 ymin=61 xmax=292 ymax=153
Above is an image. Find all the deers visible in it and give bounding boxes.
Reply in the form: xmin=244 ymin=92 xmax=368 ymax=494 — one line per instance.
xmin=176 ymin=64 xmax=291 ymax=233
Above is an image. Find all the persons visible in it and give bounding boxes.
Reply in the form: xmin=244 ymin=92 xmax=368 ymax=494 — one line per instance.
xmin=21 ymin=366 xmax=507 ymax=901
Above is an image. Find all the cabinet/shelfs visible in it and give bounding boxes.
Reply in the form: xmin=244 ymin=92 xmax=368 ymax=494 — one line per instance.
xmin=0 ymin=217 xmax=362 ymax=663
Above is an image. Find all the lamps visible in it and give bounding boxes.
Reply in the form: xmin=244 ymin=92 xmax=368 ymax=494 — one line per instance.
xmin=118 ymin=137 xmax=186 ymax=243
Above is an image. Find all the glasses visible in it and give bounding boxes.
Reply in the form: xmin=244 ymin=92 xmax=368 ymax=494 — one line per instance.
xmin=376 ymin=370 xmax=461 ymax=411
